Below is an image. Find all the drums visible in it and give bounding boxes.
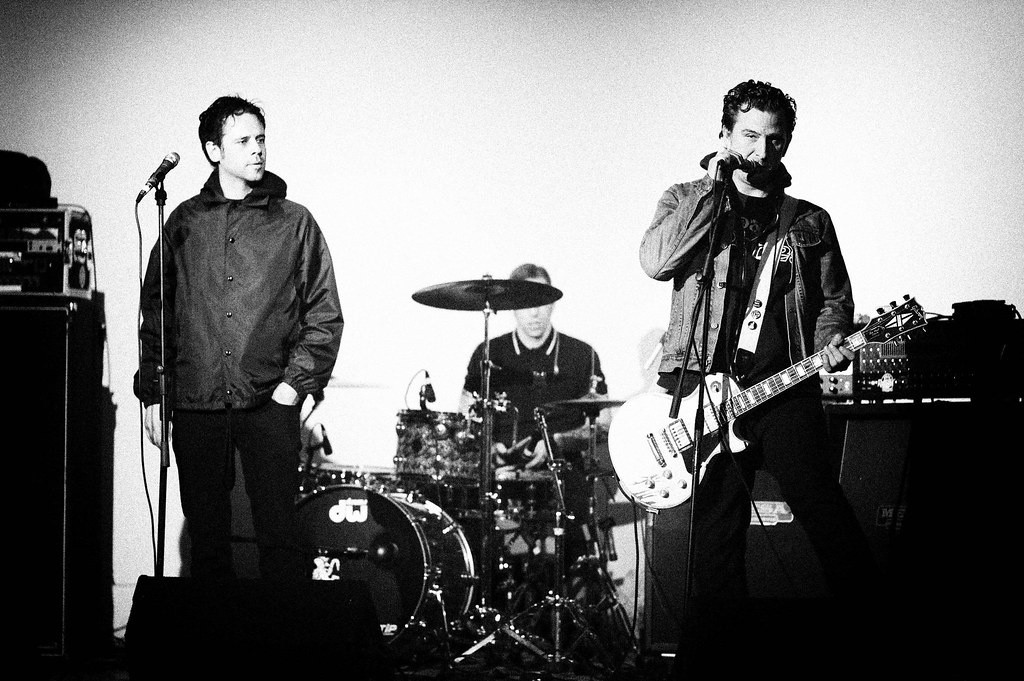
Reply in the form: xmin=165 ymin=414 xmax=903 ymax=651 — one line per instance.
xmin=403 ymin=476 xmax=484 ymax=520
xmin=289 ymin=483 xmax=476 ymax=647
xmin=395 ymin=408 xmax=483 ymax=480
xmin=495 ymin=458 xmax=563 ymax=520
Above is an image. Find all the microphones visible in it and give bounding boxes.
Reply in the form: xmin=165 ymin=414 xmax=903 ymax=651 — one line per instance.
xmin=717 ymin=158 xmax=756 ymax=172
xmin=374 ymin=543 xmax=399 ymax=566
xmin=424 ymin=369 xmax=437 ymax=402
xmin=321 ymin=423 xmax=333 ymax=456
xmin=137 ymin=152 xmax=180 ymax=203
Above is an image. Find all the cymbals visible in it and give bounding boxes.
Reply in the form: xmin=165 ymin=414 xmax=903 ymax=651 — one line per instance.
xmin=545 ymin=392 xmax=626 ymax=410
xmin=409 ymin=277 xmax=566 ymax=311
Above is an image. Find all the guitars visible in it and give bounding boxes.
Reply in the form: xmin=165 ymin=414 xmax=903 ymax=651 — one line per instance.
xmin=605 ymin=293 xmax=931 ymax=511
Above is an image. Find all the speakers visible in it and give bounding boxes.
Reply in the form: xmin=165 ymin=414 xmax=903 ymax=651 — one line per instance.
xmin=1 ymin=294 xmax=108 ymax=681
xmin=123 ymin=573 xmax=399 ymax=681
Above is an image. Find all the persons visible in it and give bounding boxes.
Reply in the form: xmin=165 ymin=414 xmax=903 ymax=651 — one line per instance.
xmin=459 ymin=263 xmax=612 ymax=472
xmin=639 ymin=79 xmax=856 ymax=681
xmin=133 ymin=97 xmax=344 ymax=580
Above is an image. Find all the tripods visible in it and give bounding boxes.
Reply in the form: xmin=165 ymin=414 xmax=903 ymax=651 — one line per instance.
xmin=434 ymin=287 xmax=646 ymax=681
